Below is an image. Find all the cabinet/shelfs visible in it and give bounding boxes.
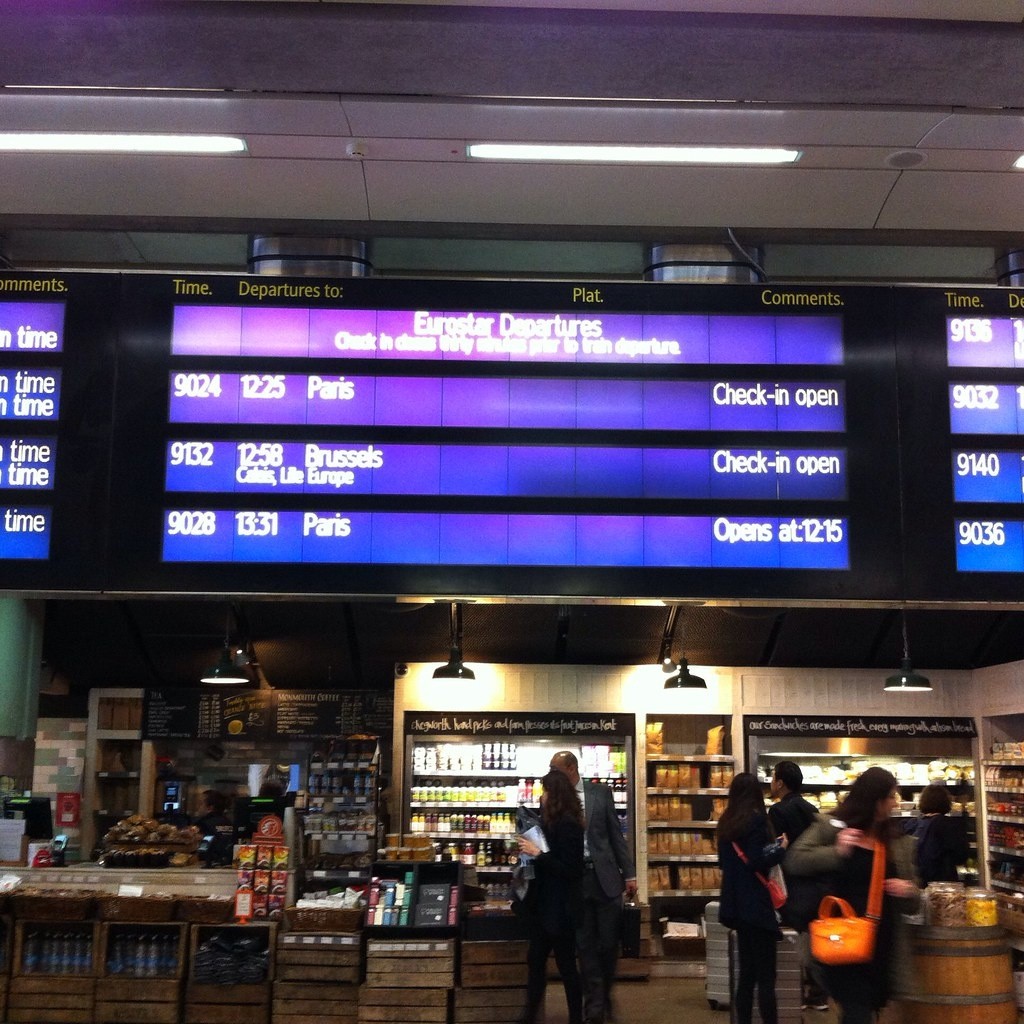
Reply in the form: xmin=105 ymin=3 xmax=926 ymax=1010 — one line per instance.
xmin=405 ymin=718 xmax=1024 ymax=959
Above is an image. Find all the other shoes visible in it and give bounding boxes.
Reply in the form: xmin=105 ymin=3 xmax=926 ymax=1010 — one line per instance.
xmin=808 ymin=1000 xmax=830 ymax=1010
xmin=801 ymin=1004 xmax=807 ymax=1009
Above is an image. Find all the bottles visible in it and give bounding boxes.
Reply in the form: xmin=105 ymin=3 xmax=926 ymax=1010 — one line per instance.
xmin=584 ymin=778 xmax=627 ymax=838
xmin=410 ymin=778 xmax=543 ymax=896
xmin=22 ymin=932 xmax=92 ymax=976
xmin=106 ymin=929 xmax=179 ymax=976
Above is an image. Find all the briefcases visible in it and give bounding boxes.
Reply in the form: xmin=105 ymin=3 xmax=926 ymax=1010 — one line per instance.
xmin=621 ymin=892 xmax=640 ymax=958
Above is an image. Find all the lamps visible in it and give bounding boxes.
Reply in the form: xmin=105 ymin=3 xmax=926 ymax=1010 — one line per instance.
xmin=201 ymin=600 xmax=251 ymax=685
xmin=662 ymin=608 xmax=707 ymax=689
xmin=432 ymin=601 xmax=476 ymax=681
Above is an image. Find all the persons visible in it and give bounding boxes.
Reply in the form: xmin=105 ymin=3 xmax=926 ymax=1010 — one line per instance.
xmin=512 ymin=772 xmax=582 ymax=1024
xmin=784 ymin=766 xmax=920 ymax=1024
xmin=550 ymin=752 xmax=638 ymax=1024
xmin=717 ymin=762 xmax=830 ymax=1024
xmin=192 ymin=789 xmax=236 ymax=848
xmin=912 ymin=784 xmax=969 ymax=889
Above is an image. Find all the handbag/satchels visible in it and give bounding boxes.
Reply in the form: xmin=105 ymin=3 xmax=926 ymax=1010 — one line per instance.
xmin=768 ymin=879 xmax=788 ymax=908
xmin=808 ymin=896 xmax=878 ymax=965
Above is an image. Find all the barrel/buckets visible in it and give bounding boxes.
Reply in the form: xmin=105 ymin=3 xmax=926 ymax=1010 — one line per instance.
xmin=891 ymin=914 xmax=1018 ymax=1023
